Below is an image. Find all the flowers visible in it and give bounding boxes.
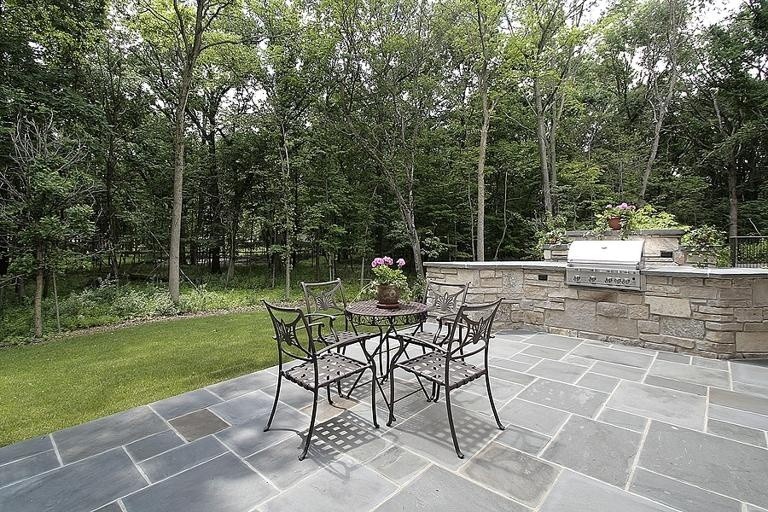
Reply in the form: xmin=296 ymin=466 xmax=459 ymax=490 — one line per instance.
xmin=586 ymin=197 xmax=638 ymax=240
xmin=368 ymin=254 xmax=414 ymax=305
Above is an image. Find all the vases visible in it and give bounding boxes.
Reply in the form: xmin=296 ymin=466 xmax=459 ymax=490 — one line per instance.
xmin=376 ymin=283 xmax=399 ymax=308
xmin=607 ymin=217 xmax=621 ymax=230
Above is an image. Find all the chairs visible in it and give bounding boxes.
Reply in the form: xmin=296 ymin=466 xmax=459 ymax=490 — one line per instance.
xmin=383 ymin=297 xmax=504 ymax=457
xmin=258 ymin=301 xmax=381 ymax=461
xmin=387 ymin=278 xmax=472 ymax=399
xmin=303 ymin=279 xmax=381 ymax=397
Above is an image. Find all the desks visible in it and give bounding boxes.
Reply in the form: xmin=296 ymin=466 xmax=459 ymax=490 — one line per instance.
xmin=340 ymin=300 xmax=430 ymax=430
